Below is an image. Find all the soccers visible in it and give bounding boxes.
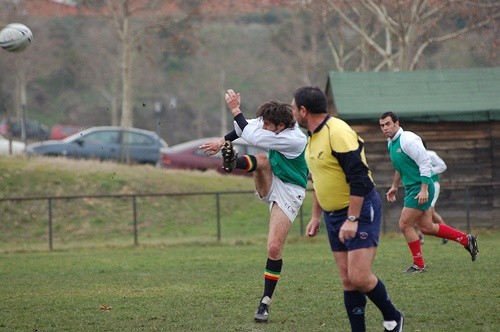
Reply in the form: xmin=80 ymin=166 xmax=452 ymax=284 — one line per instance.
xmin=0 ymin=22 xmax=33 ymax=53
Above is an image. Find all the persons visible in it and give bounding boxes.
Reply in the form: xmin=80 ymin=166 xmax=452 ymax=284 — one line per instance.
xmin=292 ymin=86 xmax=405 ymax=332
xmin=379 ymin=111 xmax=479 ymax=273
xmin=198 ymin=89 xmax=309 ymax=322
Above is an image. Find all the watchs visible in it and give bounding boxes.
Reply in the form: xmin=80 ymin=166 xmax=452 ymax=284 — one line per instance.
xmin=347 ymin=216 xmax=358 ymax=222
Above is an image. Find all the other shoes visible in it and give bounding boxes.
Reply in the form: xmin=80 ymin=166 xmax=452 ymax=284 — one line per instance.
xmin=443 ymin=238 xmax=448 ymax=243
xmin=419 ymin=235 xmax=424 ymax=244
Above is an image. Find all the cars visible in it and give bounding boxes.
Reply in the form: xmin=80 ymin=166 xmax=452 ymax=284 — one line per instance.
xmin=25 ymin=127 xmax=169 ymax=163
xmin=159 ymin=138 xmax=253 ymax=176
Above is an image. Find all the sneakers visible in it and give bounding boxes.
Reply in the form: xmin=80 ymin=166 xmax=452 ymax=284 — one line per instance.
xmin=254 ymin=296 xmax=272 ymax=322
xmin=403 ymin=264 xmax=428 ymax=273
xmin=383 ymin=311 xmax=404 ymax=332
xmin=222 ymin=141 xmax=236 ymax=172
xmin=464 ymin=234 xmax=479 ymax=262
